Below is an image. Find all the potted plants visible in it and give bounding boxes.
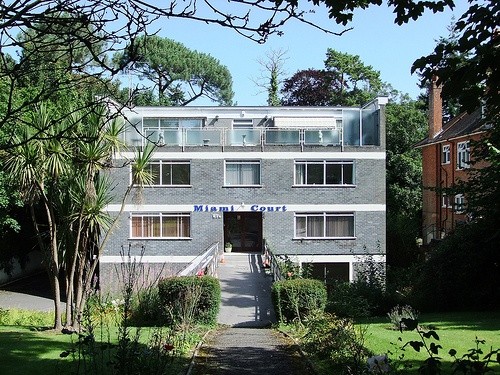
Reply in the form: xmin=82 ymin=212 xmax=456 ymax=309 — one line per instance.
xmin=225 ymin=242 xmax=232 ymax=253
